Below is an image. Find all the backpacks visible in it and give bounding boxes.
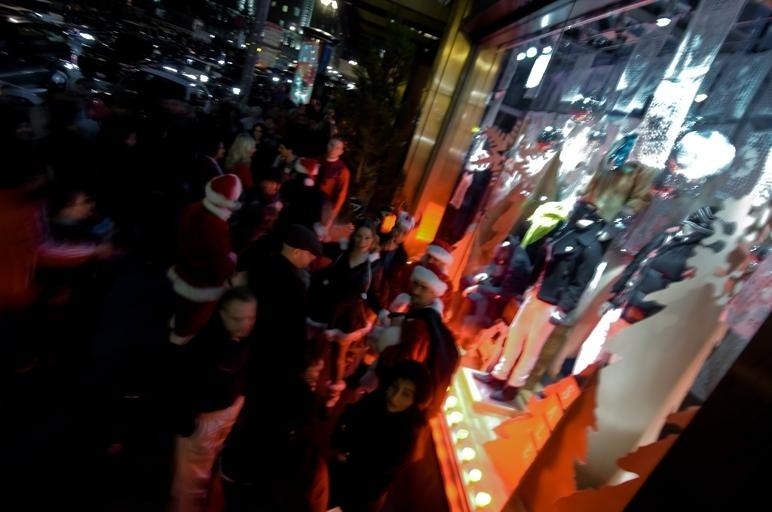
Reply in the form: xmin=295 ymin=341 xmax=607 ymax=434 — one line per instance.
xmin=408 ymin=311 xmax=460 ymax=387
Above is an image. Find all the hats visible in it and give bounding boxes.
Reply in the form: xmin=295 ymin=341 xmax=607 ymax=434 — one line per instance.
xmin=409 ymin=261 xmax=453 ymax=298
xmin=395 ymin=211 xmax=416 ymax=233
xmin=205 ymin=173 xmax=245 ymax=212
xmin=294 ymin=157 xmax=321 ymax=176
xmin=283 ymin=223 xmax=325 ymax=257
xmin=426 ymin=238 xmax=455 ymax=266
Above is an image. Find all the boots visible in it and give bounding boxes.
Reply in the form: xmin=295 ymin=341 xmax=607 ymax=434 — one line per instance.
xmin=301 ymin=360 xmax=324 ymax=393
xmin=323 ymin=379 xmax=346 ymax=417
xmin=343 ymin=360 xmax=370 ymax=387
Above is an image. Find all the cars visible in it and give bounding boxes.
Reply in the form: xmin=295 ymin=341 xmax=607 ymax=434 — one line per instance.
xmin=0 ymin=0 xmax=360 ymax=104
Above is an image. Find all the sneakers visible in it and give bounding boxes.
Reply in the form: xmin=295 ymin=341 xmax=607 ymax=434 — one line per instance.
xmin=169 ymin=315 xmax=193 ymax=345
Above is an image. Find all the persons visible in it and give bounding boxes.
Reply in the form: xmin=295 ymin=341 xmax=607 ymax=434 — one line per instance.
xmin=458 ymin=149 xmax=724 ymax=403
xmin=27 ymin=122 xmax=457 ymax=512
